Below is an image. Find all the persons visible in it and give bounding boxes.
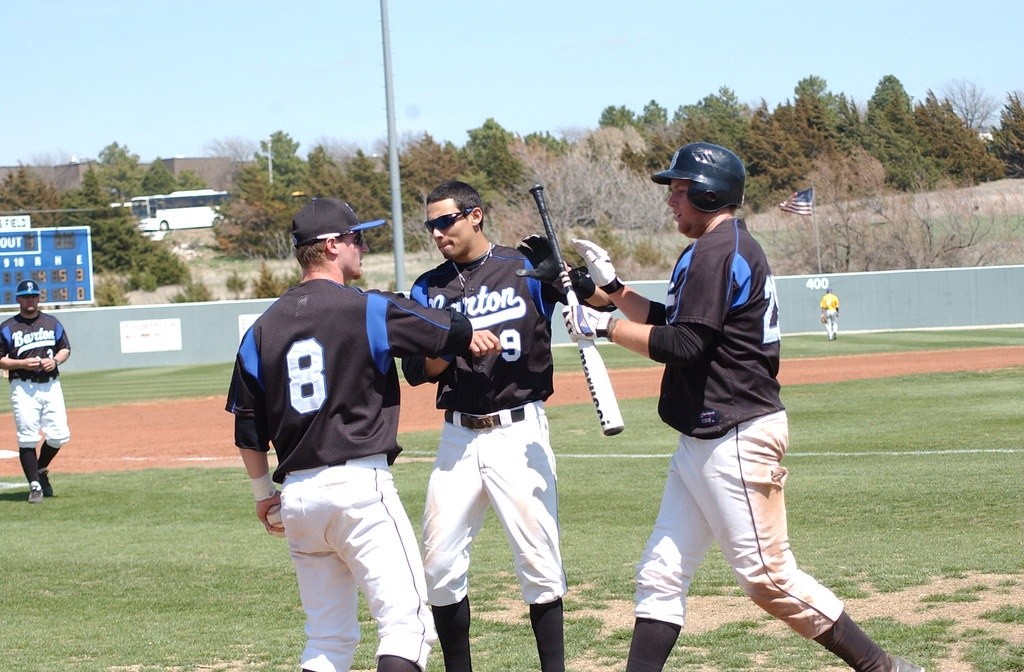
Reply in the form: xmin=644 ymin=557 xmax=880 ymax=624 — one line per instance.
xmin=224 ymin=197 xmax=473 ymax=672
xmin=401 ymin=179 xmax=618 ymax=672
xmin=570 ymin=142 xmax=923 ymax=672
xmin=0 ymin=280 xmax=71 ymax=503
xmin=820 ymin=290 xmax=839 ymax=341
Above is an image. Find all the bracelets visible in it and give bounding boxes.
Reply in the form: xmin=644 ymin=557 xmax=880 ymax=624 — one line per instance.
xmin=53 ymin=358 xmax=59 ymax=365
xmin=249 ymin=471 xmax=277 ymax=501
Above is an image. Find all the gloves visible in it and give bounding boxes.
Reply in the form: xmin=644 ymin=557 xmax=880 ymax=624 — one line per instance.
xmin=514 ymin=234 xmax=588 ymax=291
xmin=561 ymin=305 xmax=617 ymax=342
xmin=570 ymin=238 xmax=620 ymax=291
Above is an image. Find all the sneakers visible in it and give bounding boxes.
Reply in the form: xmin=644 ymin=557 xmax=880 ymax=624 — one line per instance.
xmin=28 ymin=481 xmax=44 ymax=503
xmin=37 ymin=468 xmax=53 ymax=497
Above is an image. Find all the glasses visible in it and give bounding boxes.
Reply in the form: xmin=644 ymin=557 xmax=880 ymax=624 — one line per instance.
xmin=314 ymin=230 xmax=363 ymax=245
xmin=424 ymin=209 xmax=474 ymax=234
xmin=18 ymin=294 xmax=38 ymax=299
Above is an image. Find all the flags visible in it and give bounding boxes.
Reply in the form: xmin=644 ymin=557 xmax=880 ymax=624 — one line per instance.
xmin=779 ymin=188 xmax=812 ymax=215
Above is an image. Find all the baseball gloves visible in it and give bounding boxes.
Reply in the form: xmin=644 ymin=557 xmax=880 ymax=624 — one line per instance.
xmin=820 ymin=314 xmax=827 ymax=323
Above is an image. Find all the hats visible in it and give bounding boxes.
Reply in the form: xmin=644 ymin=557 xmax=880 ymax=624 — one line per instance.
xmin=16 ymin=280 xmax=41 ymax=296
xmin=292 ymin=198 xmax=385 ymax=250
xmin=827 ymin=289 xmax=832 ymax=294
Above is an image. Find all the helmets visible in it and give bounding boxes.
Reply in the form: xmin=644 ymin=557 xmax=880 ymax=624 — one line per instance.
xmin=651 ymin=142 xmax=746 ymax=212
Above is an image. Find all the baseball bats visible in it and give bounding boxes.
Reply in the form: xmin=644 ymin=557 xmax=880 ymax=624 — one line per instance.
xmin=527 ymin=182 xmax=626 ymax=437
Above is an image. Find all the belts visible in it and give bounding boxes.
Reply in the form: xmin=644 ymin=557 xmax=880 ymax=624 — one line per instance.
xmin=20 ymin=376 xmax=56 ymax=383
xmin=444 ymin=408 xmax=525 ymax=431
